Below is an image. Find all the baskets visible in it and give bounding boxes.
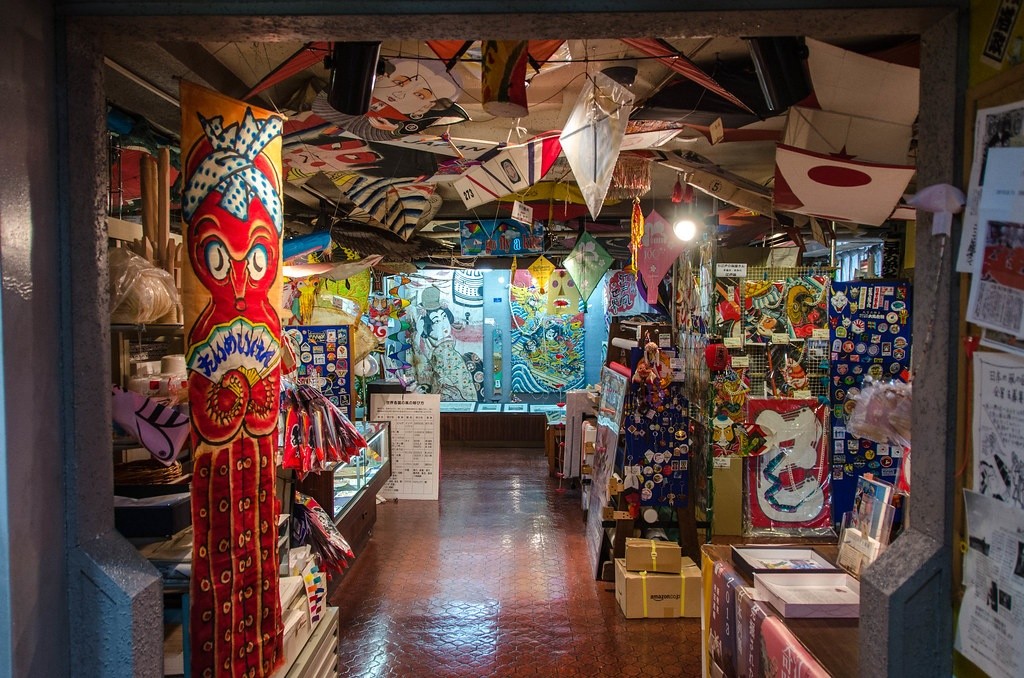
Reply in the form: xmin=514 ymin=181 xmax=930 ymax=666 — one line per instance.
xmin=113 ymin=459 xmax=183 ymax=483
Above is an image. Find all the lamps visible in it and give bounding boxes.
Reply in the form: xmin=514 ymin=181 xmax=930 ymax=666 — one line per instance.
xmin=672 ymin=202 xmax=697 ymax=241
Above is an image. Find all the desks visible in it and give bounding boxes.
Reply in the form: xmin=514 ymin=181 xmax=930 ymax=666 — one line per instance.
xmin=697 ymin=542 xmax=861 ymax=678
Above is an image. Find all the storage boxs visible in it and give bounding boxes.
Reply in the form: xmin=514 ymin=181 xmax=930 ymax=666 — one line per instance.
xmin=752 ymin=572 xmax=860 ymax=619
xmin=614 ymin=557 xmax=701 ymax=619
xmin=728 ymin=544 xmax=842 ymax=582
xmin=624 ymin=537 xmax=682 ymax=574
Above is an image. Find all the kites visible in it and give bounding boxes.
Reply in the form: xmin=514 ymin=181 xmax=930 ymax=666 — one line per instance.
xmin=178 ymin=69 xmax=379 ymax=678
xmin=272 ymin=40 xmax=687 ymax=313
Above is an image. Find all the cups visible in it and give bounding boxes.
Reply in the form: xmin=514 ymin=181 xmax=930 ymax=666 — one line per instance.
xmin=128 ymin=373 xmax=178 ymax=395
